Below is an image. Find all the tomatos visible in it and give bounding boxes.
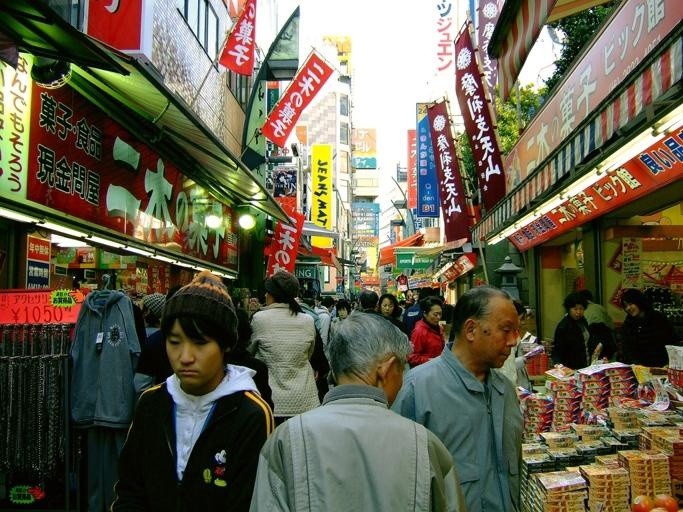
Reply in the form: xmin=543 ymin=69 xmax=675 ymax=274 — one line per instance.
xmin=632 ymin=493 xmax=678 ymax=512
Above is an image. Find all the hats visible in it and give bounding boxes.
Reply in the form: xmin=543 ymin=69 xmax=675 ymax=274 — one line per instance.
xmin=142 ymin=293 xmax=167 ymax=319
xmin=162 ymin=270 xmax=240 ymax=344
xmin=264 ymin=270 xmax=299 ymax=298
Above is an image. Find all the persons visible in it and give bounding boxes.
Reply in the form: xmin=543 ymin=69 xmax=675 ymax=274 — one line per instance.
xmin=554 ymin=289 xmax=669 ymax=370
xmin=106 ymin=267 xmax=535 ymax=511
